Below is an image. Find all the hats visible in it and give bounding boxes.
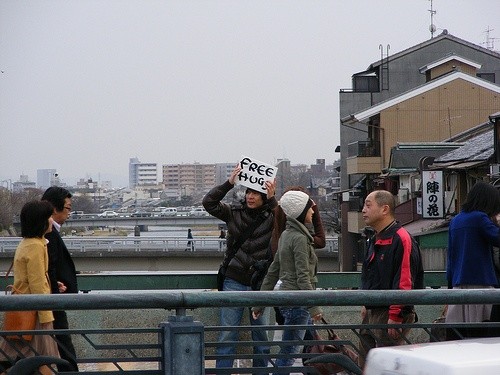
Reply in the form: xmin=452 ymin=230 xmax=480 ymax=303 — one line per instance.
xmin=278 ymin=191 xmax=310 ymax=220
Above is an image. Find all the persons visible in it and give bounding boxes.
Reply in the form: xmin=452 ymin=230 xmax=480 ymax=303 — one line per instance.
xmin=202 ymin=163 xmax=326 ymax=375
xmin=217 ymin=226 xmax=226 ymax=252
xmin=0 ymin=185 xmax=79 ymax=375
xmin=355 ymin=190 xmax=419 ymax=372
xmin=134 ymin=226 xmax=142 ymax=250
xmin=184 ymin=229 xmax=194 ymax=252
xmin=430 ymin=179 xmax=499 ymax=342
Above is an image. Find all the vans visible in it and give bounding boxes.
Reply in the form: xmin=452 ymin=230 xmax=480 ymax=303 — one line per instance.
xmin=67 ymin=211 xmax=83 ymax=219
xmin=160 ymin=208 xmax=177 ymax=217
xmin=190 ymin=208 xmax=207 ymax=217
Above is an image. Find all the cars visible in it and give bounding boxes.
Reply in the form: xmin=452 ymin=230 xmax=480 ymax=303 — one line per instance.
xmin=98 ymin=211 xmax=118 ymax=218
xmin=130 ymin=209 xmax=151 ymax=217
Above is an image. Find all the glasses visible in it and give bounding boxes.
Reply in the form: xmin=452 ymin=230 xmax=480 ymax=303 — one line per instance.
xmin=64 ymin=207 xmax=71 ymax=211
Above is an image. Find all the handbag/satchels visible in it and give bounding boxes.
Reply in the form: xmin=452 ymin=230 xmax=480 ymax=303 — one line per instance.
xmin=4 ymin=285 xmax=37 ymax=343
xmin=217 ymin=266 xmax=227 ymax=291
xmin=303 ymin=317 xmax=359 ymax=372
xmin=430 ymin=305 xmax=448 ymax=342
